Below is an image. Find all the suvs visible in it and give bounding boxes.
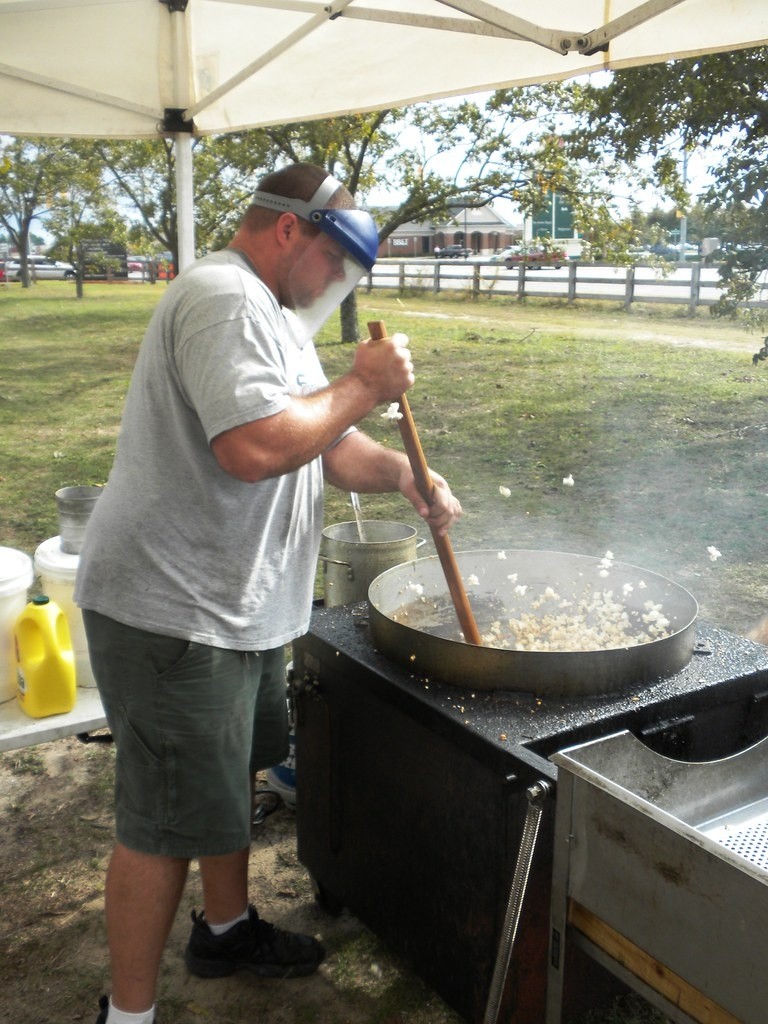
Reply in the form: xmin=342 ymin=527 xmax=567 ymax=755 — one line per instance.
xmin=3 ymin=254 xmax=76 ymax=281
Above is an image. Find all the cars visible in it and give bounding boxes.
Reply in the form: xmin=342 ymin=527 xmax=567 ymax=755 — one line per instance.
xmin=490 ymin=246 xmax=523 ymax=262
xmin=649 ymin=242 xmax=677 ymax=255
xmin=127 ymin=257 xmax=142 ymax=269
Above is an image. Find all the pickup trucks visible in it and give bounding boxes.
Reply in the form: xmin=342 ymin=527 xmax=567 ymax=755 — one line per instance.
xmin=503 ymin=245 xmax=568 ymax=270
xmin=435 ymin=244 xmax=472 ymax=259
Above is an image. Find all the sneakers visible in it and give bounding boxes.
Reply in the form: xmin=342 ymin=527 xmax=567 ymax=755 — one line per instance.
xmin=184 ymin=903 xmax=326 ymax=978
xmin=95 ymin=994 xmax=157 ymax=1024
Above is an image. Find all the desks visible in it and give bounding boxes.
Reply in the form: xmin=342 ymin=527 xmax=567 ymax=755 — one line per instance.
xmin=0 ymin=685 xmax=111 ymax=753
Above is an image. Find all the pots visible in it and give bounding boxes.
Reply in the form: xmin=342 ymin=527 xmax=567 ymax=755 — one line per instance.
xmin=317 ymin=520 xmax=427 ymax=610
xmin=354 ymin=549 xmax=711 ymax=694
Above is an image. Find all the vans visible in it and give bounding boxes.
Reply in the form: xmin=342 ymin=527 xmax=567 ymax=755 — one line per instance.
xmin=550 ymin=239 xmax=602 ymax=258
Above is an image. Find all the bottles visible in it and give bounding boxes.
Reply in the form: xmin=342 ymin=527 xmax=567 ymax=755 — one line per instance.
xmin=12 ymin=594 xmax=75 ymax=718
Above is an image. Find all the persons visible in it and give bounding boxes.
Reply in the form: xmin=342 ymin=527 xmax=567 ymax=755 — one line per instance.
xmin=74 ymin=162 xmax=462 ymax=1024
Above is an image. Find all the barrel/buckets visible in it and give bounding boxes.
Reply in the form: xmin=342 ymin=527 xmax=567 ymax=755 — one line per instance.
xmin=0 ymin=546 xmax=35 ymax=703
xmin=35 ymin=534 xmax=97 ymax=687
xmin=54 ymin=485 xmax=105 ymax=555
